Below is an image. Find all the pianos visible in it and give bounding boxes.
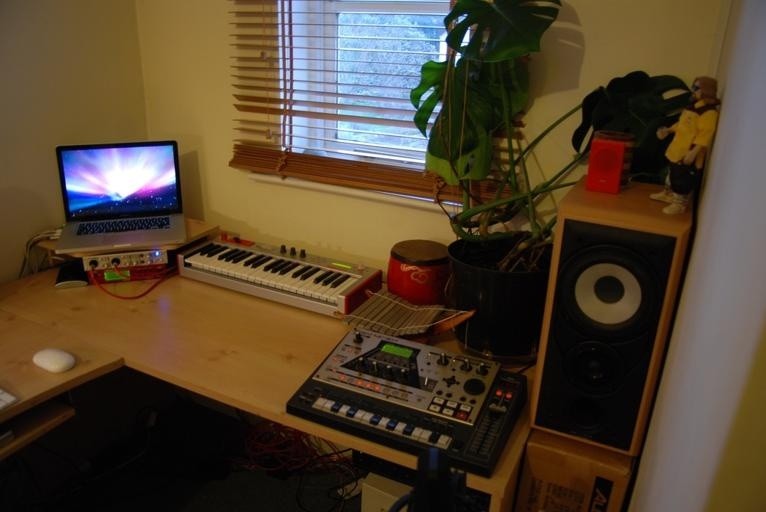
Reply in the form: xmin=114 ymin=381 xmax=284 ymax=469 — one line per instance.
xmin=169 ymin=221 xmax=386 ymax=319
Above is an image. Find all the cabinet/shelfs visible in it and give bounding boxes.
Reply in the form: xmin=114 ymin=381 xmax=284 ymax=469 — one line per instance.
xmin=511 ymin=427 xmax=635 ymax=512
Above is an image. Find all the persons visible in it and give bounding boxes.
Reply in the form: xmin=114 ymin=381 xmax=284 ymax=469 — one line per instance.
xmin=651 ymin=77 xmax=721 ymax=212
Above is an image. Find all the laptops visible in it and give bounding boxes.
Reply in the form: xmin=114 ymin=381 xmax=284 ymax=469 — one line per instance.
xmin=53 ymin=140 xmax=185 ymax=253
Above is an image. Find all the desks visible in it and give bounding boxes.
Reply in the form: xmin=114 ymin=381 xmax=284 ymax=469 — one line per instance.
xmin=1 ymin=261 xmax=527 ymax=512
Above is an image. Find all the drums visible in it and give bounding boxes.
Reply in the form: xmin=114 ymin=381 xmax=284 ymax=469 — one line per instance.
xmin=387 ymin=238 xmax=450 ymax=307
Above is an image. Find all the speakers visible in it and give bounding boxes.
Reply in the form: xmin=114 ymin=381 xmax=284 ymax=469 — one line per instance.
xmin=529 ymin=175 xmax=694 ymax=457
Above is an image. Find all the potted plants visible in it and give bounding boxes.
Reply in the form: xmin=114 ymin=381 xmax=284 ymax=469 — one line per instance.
xmin=410 ymin=0 xmax=693 ymax=361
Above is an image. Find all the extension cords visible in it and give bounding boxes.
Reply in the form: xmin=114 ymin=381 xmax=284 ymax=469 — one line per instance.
xmin=82 ymin=249 xmax=169 ymax=270
